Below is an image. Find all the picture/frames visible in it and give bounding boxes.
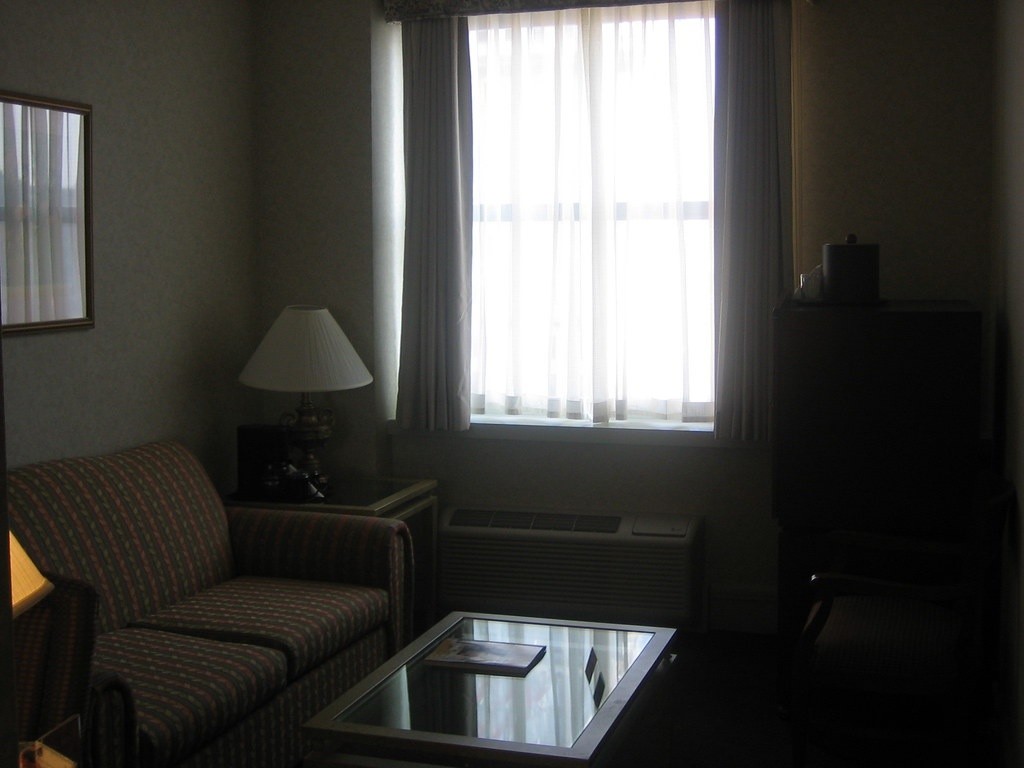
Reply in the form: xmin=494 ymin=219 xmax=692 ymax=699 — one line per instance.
xmin=0 ymin=90 xmax=96 ymax=339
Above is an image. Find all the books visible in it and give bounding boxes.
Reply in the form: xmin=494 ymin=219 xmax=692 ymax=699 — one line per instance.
xmin=423 ymin=636 xmax=546 ymax=678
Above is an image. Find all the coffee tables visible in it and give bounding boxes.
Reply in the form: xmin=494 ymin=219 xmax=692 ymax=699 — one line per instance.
xmin=301 ymin=611 xmax=678 ymax=768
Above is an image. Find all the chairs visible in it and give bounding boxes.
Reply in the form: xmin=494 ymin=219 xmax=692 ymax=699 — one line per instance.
xmin=789 ymin=471 xmax=1017 ymax=768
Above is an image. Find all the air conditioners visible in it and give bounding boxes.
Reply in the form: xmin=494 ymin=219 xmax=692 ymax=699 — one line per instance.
xmin=438 ymin=507 xmax=704 ymax=623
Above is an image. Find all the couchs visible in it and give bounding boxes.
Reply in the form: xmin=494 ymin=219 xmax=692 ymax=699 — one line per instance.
xmin=5 ymin=438 xmax=416 ymax=768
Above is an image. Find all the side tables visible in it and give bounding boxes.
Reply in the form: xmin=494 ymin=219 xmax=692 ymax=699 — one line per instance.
xmin=225 ymin=476 xmax=443 ymax=627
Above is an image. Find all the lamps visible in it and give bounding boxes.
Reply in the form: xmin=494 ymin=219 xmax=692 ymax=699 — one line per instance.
xmin=236 ymin=304 xmax=373 ymax=499
